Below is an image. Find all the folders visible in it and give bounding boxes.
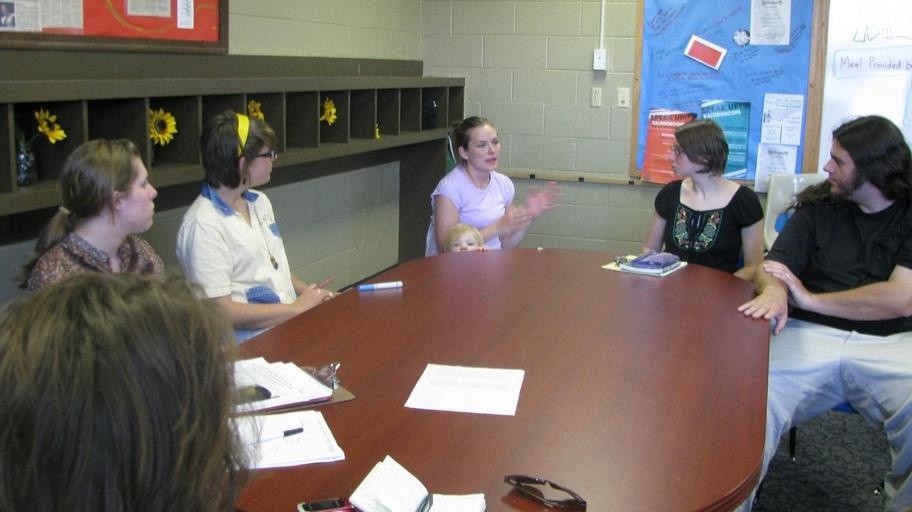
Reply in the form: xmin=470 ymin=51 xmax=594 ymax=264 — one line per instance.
xmin=231 ymin=357 xmax=356 ymax=417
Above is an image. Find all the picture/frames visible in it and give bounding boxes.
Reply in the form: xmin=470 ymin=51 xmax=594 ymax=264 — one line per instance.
xmin=0 ymin=0 xmax=229 ymax=58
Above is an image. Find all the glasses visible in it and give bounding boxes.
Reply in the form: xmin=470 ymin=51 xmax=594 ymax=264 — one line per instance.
xmin=672 ymin=146 xmax=685 ymax=157
xmin=257 ymin=150 xmax=277 ymax=161
xmin=504 ymin=474 xmax=587 ymax=512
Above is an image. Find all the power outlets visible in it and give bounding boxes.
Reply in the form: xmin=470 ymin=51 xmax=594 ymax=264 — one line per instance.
xmin=618 ymin=88 xmax=630 ymax=108
xmin=590 ymin=87 xmax=602 ymax=106
xmin=593 ymin=49 xmax=607 ymax=71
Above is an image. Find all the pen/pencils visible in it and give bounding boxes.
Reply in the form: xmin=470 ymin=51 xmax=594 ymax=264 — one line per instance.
xmin=258 ymin=428 xmax=304 ymax=444
xmin=316 ymin=276 xmax=337 ymax=289
xmin=419 ymin=496 xmax=429 ymax=512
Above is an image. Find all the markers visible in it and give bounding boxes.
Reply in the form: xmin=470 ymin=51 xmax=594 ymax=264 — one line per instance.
xmin=357 ymin=281 xmax=403 ymax=291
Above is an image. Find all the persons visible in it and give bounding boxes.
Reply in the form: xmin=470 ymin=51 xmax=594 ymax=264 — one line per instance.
xmin=640 ymin=119 xmax=764 ymax=281
xmin=426 ymin=116 xmax=565 ymax=256
xmin=19 ymin=138 xmax=165 ymax=292
xmin=175 ymin=110 xmax=343 ymax=349
xmin=2 ymin=272 xmax=254 ymax=510
xmin=734 ymin=115 xmax=912 ymax=511
xmin=443 ymin=222 xmax=488 ymax=252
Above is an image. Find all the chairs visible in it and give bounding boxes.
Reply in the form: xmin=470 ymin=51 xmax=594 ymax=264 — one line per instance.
xmin=789 ymin=402 xmax=860 ymax=459
xmin=763 ymin=172 xmax=830 ymax=252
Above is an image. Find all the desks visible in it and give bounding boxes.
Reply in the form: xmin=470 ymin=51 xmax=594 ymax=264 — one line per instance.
xmin=222 ymin=247 xmax=770 ymax=512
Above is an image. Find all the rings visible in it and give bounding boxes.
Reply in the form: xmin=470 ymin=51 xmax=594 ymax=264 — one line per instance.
xmin=316 ymin=288 xmax=320 ymax=294
xmin=786 ymin=273 xmax=792 ymax=279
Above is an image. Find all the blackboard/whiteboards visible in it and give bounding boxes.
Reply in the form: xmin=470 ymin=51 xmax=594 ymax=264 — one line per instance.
xmin=812 ymin=0 xmax=911 ymax=174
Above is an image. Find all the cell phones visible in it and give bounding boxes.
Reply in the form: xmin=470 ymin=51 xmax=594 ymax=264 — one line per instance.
xmin=297 ymin=498 xmax=350 ymax=511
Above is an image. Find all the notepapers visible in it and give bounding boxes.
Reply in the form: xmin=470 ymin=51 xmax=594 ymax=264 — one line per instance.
xmin=684 ymin=35 xmax=727 ymax=71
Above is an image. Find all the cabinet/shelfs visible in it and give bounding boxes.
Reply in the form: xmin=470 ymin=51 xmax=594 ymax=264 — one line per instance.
xmin=1 ymin=47 xmax=465 ymax=246
